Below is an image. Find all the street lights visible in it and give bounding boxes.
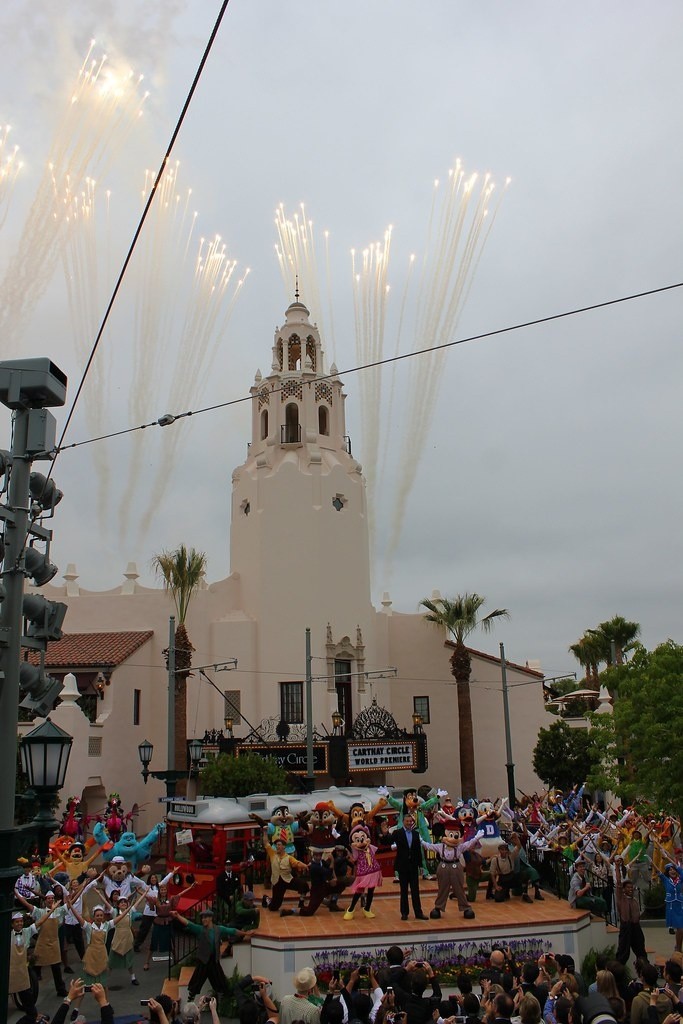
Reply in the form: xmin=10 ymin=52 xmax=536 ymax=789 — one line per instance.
xmin=138 ymin=738 xmax=203 ymax=850
xmin=0 ymin=357 xmax=75 ymax=1024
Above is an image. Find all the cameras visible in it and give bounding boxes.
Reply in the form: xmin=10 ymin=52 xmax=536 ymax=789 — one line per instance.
xmin=545 ymin=953 xmax=549 ymax=959
xmin=449 ymin=994 xmax=459 ymax=1002
xmin=454 ymin=1016 xmax=465 ymax=1024
xmin=359 ymin=968 xmax=368 ymax=976
xmin=140 ymin=1000 xmax=151 ymax=1006
xmin=492 ymin=944 xmax=508 ymax=959
xmin=394 ymin=1014 xmax=402 ymax=1020
xmin=205 ymin=997 xmax=211 ymax=1003
xmin=251 ymin=985 xmax=262 ymax=991
xmin=658 ymin=988 xmax=665 ymax=994
xmin=38 ymin=1014 xmax=50 ymax=1024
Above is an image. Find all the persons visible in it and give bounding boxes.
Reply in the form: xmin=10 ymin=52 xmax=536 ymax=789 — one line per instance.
xmin=11 ymin=789 xmax=683 ymax=1024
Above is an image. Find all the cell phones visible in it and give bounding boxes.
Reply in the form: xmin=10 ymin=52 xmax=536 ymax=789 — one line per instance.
xmin=415 ymin=962 xmax=425 ymax=968
xmin=489 ymin=992 xmax=496 ymax=1002
xmin=333 ymin=970 xmax=339 ymax=982
xmin=561 ymin=982 xmax=566 ymax=994
xmin=387 ymin=987 xmax=393 ymax=995
xmin=82 ymin=986 xmax=93 ymax=993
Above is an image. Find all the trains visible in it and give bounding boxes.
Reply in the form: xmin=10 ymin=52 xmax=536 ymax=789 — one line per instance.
xmin=163 ymin=784 xmax=427 ymax=914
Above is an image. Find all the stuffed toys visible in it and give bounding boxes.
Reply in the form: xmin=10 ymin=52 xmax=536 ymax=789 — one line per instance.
xmin=48 ymin=840 xmax=114 ymax=883
xmin=297 ymin=800 xmax=343 ymax=885
xmin=326 ymin=797 xmax=388 ymax=829
xmin=94 ymin=820 xmax=167 ymax=873
xmin=419 ymin=821 xmax=484 ymax=920
xmin=48 ymin=834 xmax=94 ymax=858
xmin=31 ymin=861 xmax=62 ymax=877
xmin=473 ymin=796 xmax=509 ymax=860
xmin=343 ymin=824 xmax=385 ymax=920
xmin=438 ymin=803 xmax=495 ymax=900
xmin=247 ymin=802 xmax=313 ymax=861
xmin=377 ymin=784 xmax=449 ymax=883
xmin=86 ymin=856 xmax=155 ymax=902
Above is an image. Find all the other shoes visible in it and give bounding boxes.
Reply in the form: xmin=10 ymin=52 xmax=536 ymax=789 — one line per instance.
xmin=280 ymin=908 xmax=292 ymax=917
xmin=63 ymin=966 xmax=75 ymax=974
xmin=449 ymin=891 xmax=457 ymax=900
xmin=400 ymin=912 xmax=408 ymax=920
xmin=484 ymin=893 xmax=545 ymax=903
xmin=360 ymin=895 xmax=368 ymax=907
xmin=414 ymin=910 xmax=428 ymax=921
xmin=143 ymin=962 xmax=149 ymax=971
xmin=131 ymin=979 xmax=140 ymax=986
xmin=260 ymin=894 xmax=269 ymax=908
xmin=329 ymin=901 xmax=344 ymax=912
xmin=133 ymin=945 xmax=158 ymax=953
xmin=56 ymin=990 xmax=69 ymax=997
xmin=669 ymin=928 xmax=676 ymax=935
xmin=297 ymin=899 xmax=306 ymax=908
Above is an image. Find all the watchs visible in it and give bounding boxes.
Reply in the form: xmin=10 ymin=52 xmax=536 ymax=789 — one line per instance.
xmin=63 ymin=997 xmax=71 ymax=1003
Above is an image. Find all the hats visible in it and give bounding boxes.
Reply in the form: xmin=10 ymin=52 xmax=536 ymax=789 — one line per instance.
xmin=12 ymin=912 xmax=23 ymax=919
xmin=632 ymin=830 xmax=644 ymax=839
xmin=242 ymin=891 xmax=255 ymax=900
xmin=313 ymin=849 xmax=323 ymax=855
xmin=291 ymin=967 xmax=317 ymax=991
xmin=199 ymin=909 xmax=215 ymax=916
xmin=574 ymin=860 xmax=586 ymax=867
xmin=45 ymin=890 xmax=55 ymax=896
xmin=91 ymin=885 xmax=128 ymax=913
